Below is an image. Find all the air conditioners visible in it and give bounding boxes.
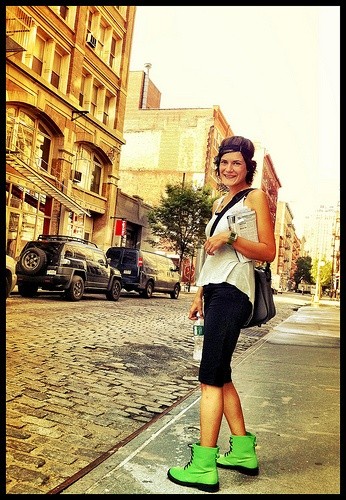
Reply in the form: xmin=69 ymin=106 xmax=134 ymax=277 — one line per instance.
xmin=87 ymin=33 xmax=96 ymax=48
xmin=72 ymin=170 xmax=82 ymax=182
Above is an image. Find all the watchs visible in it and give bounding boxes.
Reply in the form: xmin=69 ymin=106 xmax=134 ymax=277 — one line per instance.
xmin=226 ymin=230 xmax=238 ymax=246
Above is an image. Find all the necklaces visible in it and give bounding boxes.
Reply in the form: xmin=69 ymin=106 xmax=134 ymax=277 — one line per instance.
xmin=220 ymin=205 xmax=223 ymax=209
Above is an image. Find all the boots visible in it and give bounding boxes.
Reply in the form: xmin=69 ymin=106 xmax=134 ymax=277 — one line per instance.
xmin=167 ymin=443 xmax=220 ymax=493
xmin=218 ymin=433 xmax=258 ymax=476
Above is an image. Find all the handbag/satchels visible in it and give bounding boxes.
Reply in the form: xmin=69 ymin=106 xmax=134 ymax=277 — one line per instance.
xmin=240 ymin=268 xmax=277 ymax=329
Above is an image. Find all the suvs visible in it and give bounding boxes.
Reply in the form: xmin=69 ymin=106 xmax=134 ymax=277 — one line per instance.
xmin=15 ymin=234 xmax=123 ymax=302
xmin=106 ymin=247 xmax=182 ymax=299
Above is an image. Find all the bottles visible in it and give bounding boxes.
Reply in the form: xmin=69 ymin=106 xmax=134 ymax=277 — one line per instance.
xmin=192 ymin=311 xmax=204 ymax=361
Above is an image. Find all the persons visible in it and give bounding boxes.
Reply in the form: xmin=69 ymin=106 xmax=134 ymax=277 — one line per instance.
xmin=168 ymin=135 xmax=276 ymax=493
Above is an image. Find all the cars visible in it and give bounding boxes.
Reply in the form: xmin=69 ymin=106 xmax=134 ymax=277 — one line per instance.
xmin=6 ymin=255 xmax=18 ymax=300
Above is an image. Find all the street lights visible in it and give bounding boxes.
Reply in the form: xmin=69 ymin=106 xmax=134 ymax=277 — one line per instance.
xmin=314 ymin=205 xmax=335 ymax=302
